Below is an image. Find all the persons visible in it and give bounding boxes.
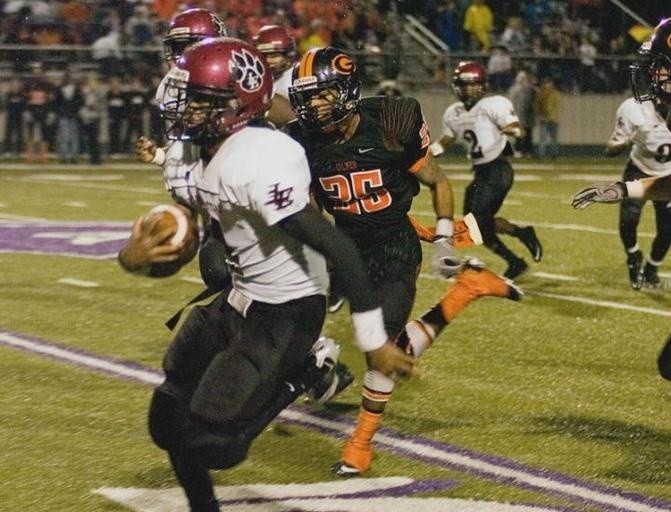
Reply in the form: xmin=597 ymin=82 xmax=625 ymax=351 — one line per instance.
xmin=603 ymin=42 xmax=671 ymax=292
xmin=121 ymin=36 xmax=416 ymax=512
xmin=570 ymin=19 xmax=671 ymax=381
xmin=427 ymin=60 xmax=544 ymax=280
xmin=0 ymin=1 xmax=670 ymax=168
xmin=288 ymin=45 xmax=524 ymax=477
xmin=247 ymin=25 xmax=346 ymax=314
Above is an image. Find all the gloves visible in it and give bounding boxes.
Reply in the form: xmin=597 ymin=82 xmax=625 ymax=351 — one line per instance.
xmin=572 ymin=180 xmax=626 ymax=209
xmin=432 ymin=238 xmax=464 ymax=275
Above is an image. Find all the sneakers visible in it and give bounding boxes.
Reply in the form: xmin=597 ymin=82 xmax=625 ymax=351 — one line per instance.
xmin=304 ymin=338 xmax=354 ymax=407
xmin=460 ymin=257 xmax=524 ymax=302
xmin=518 ymin=225 xmax=542 ymax=261
xmin=338 ymin=445 xmax=369 ymax=475
xmin=506 ymin=260 xmax=528 ymax=278
xmin=628 ymin=249 xmax=661 ymax=290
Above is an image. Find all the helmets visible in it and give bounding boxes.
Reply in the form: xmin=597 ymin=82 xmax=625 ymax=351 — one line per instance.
xmin=252 ymin=23 xmax=296 ymax=75
xmin=629 ymin=17 xmax=670 ymax=121
xmin=287 ymin=46 xmax=362 ymax=133
xmin=164 ymin=8 xmax=229 ymax=64
xmin=450 ymin=59 xmax=487 ymax=106
xmin=160 ymin=35 xmax=273 ymax=145
xmin=377 ymin=79 xmax=404 ymax=97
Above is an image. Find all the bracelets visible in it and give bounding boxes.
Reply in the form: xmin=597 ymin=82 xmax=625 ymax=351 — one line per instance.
xmin=623 ymin=179 xmax=644 ymax=203
xmin=352 ymin=309 xmax=389 ymax=354
xmin=431 ymin=219 xmax=455 ymax=239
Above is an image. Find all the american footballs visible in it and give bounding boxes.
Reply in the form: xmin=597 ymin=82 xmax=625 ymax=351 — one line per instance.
xmin=142 ymin=203 xmax=198 ymax=265
